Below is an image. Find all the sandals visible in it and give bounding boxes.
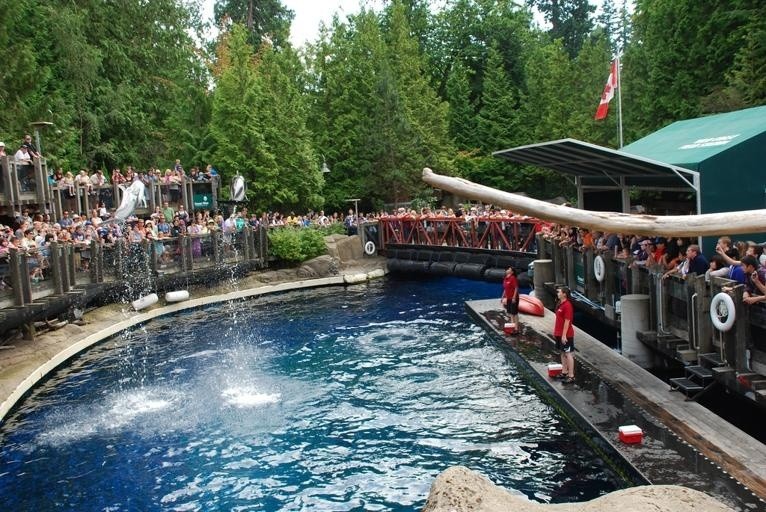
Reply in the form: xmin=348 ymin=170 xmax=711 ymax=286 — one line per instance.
xmin=561 ymin=376 xmax=575 ymax=383
xmin=556 ymin=373 xmax=568 ymax=378
xmin=509 ymin=330 xmax=521 ymax=335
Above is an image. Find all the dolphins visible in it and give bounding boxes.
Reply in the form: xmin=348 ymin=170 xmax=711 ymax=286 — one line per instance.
xmin=227 ymin=170 xmax=250 ymax=204
xmin=95 ymin=180 xmax=147 ymax=228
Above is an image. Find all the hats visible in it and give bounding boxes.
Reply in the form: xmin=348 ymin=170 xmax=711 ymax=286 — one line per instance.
xmin=71 ymin=214 xmax=80 ymax=220
xmin=144 ymin=219 xmax=154 ymax=227
xmin=0 ymin=141 xmax=5 ymax=147
xmin=652 ymin=237 xmax=666 ymax=244
xmin=638 ymin=239 xmax=651 ymax=245
xmin=20 ymin=144 xmax=28 ymax=148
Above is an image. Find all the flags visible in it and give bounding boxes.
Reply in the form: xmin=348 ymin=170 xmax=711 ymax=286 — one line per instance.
xmin=595 ymin=59 xmax=618 ymax=121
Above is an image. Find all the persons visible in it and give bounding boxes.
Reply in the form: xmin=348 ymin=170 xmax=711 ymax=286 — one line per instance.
xmin=553 ymin=287 xmax=576 ymax=385
xmin=383 ymin=204 xmax=543 ymax=253
xmin=501 ymin=266 xmax=520 ymax=335
xmin=537 ymin=201 xmax=765 ymax=305
xmin=0 ymin=136 xmax=220 ymax=289
xmin=218 ymin=207 xmax=387 ymax=249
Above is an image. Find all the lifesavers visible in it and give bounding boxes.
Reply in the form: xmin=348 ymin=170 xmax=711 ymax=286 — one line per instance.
xmin=710 ymin=292 xmax=735 ymax=331
xmin=365 ymin=241 xmax=375 ymax=255
xmin=594 ymin=256 xmax=605 ymax=281
